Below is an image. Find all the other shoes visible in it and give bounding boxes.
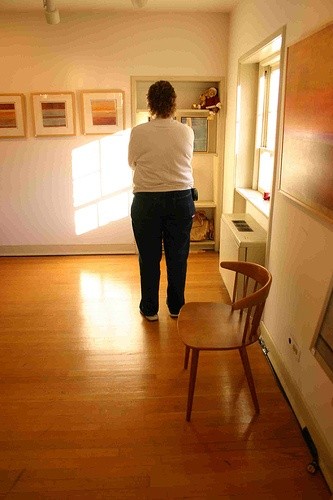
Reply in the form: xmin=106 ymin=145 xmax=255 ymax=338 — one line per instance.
xmin=168 ymin=308 xmax=182 ymax=317
xmin=139 ymin=302 xmax=158 ymax=321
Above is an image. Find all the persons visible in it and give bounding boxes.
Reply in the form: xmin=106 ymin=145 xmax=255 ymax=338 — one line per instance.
xmin=128 ymin=81 xmax=198 ymax=321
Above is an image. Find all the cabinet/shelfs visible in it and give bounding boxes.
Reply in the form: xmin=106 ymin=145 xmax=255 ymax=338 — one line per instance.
xmin=190 ymin=200 xmax=218 ymax=253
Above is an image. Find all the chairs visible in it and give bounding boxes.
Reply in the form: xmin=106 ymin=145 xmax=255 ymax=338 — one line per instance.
xmin=177 ymin=260 xmax=273 ymax=424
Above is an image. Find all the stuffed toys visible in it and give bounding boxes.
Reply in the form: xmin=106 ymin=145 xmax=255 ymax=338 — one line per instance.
xmin=193 ymin=87 xmax=221 ymax=120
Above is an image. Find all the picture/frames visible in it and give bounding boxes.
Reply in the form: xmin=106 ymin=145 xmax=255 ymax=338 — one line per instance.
xmin=0 ymin=89 xmax=126 ymax=140
